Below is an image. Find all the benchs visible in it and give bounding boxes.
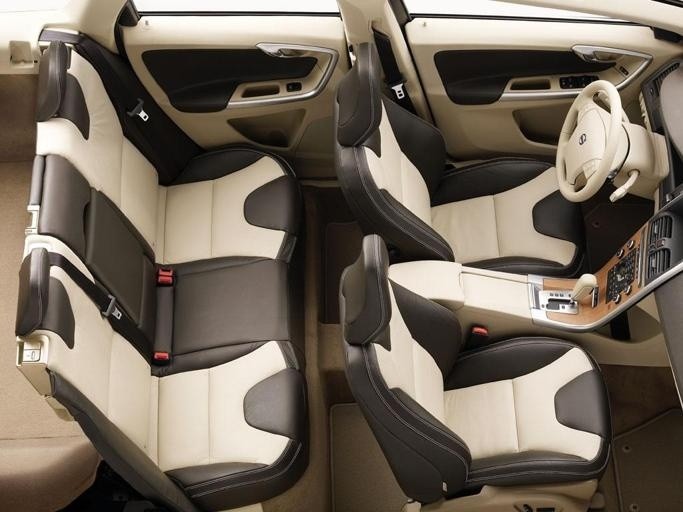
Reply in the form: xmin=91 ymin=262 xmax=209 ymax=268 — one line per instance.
xmin=0 ymin=159 xmax=105 ymax=511
xmin=12 ymin=35 xmax=312 ymax=511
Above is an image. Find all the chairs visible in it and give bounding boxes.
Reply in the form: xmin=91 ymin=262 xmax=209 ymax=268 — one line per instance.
xmin=335 ymin=42 xmax=586 ymax=279
xmin=337 ymin=235 xmax=612 ymax=507
